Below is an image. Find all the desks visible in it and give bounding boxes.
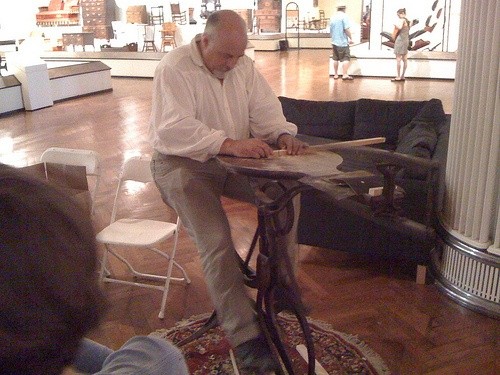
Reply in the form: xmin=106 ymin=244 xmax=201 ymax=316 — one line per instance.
xmin=176 ymin=147 xmax=343 ymax=375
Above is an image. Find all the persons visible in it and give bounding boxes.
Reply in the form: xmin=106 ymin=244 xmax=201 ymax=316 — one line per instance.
xmin=365 ymin=6 xmax=370 ymax=27
xmin=391 ymin=9 xmax=409 ymax=82
xmin=330 ymin=5 xmax=353 ymax=79
xmin=150 ymin=10 xmax=311 ymax=375
xmin=0 ymin=164 xmax=188 ymax=375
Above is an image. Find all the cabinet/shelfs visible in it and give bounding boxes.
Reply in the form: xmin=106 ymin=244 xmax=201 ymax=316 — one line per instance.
xmin=233 ymin=9 xmax=253 ymax=33
xmin=254 ymin=0 xmax=282 ymax=33
xmin=62 ymin=33 xmax=95 ymax=51
xmin=81 ymin=0 xmax=115 ymax=40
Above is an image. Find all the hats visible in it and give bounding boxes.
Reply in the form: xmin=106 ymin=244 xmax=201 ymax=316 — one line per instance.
xmin=337 ymin=5 xmax=347 ymax=8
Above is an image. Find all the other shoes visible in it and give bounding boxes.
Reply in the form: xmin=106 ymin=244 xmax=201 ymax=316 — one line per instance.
xmin=280 ymin=283 xmax=311 ymax=315
xmin=392 ymin=77 xmax=405 ymax=81
xmin=342 ymin=76 xmax=353 ymax=80
xmin=334 ymin=75 xmax=338 ymax=79
xmin=237 ymin=340 xmax=278 ymax=375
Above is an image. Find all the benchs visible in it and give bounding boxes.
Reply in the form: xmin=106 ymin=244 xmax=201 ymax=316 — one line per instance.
xmin=35 ymin=0 xmax=82 ymax=27
xmin=277 ymin=96 xmax=452 ymax=285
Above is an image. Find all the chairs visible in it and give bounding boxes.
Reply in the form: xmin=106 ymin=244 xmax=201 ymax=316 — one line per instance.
xmin=159 ymin=23 xmax=177 ymax=53
xmin=142 ymin=25 xmax=158 ymax=52
xmin=318 ymin=10 xmax=330 ymax=33
xmin=39 ymin=147 xmax=110 ymax=277
xmin=147 ymin=6 xmax=164 ymax=25
xmin=170 ymin=3 xmax=186 ymax=25
xmin=96 ymin=157 xmax=190 ymax=319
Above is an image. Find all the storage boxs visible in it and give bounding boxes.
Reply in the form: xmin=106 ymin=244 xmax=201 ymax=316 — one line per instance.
xmin=19 ymin=162 xmax=93 ymax=217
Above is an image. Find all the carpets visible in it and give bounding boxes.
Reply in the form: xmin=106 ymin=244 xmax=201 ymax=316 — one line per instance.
xmin=148 ymin=310 xmax=391 ymax=375
